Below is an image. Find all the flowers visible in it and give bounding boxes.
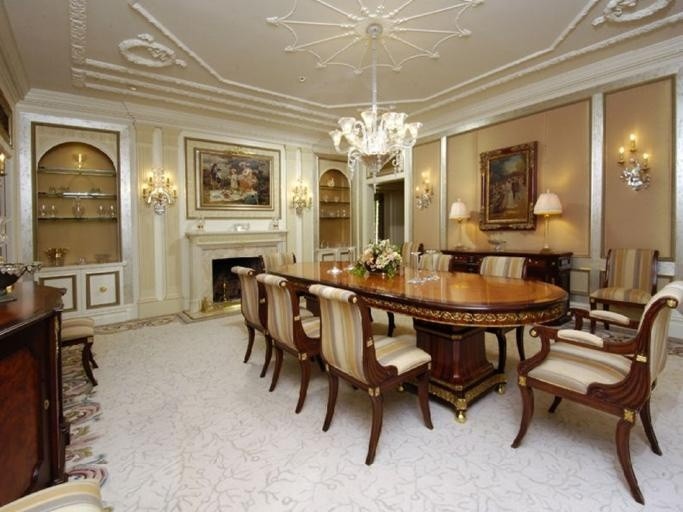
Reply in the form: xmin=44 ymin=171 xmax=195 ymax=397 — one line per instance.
xmin=355 ymin=237 xmax=402 ymax=277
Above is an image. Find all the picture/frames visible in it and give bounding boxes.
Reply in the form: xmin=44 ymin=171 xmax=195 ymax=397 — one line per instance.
xmin=193 ymin=147 xmax=276 ymax=212
xmin=480 ymin=140 xmax=538 ymax=232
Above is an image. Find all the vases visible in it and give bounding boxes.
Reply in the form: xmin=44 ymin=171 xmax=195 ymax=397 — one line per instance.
xmin=365 ymin=258 xmax=389 ymax=274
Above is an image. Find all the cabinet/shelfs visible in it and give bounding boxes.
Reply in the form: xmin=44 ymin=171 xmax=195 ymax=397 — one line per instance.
xmin=37 ymin=269 xmax=122 ymax=313
xmin=441 ymin=246 xmax=575 ymax=327
xmin=30 ymin=122 xmax=122 ymax=263
xmin=321 ymin=251 xmax=350 ymax=262
xmin=320 ymin=168 xmax=353 ymax=249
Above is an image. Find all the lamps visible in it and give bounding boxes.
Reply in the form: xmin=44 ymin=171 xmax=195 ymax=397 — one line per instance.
xmin=415 ymin=171 xmax=434 ymax=211
xmin=533 ymin=189 xmax=563 ymax=253
xmin=617 ymin=134 xmax=652 ymax=192
xmin=448 ymin=198 xmax=471 ymax=249
xmin=289 ymin=187 xmax=313 ymax=216
xmin=328 ymin=24 xmax=423 ymax=183
xmin=143 ymin=173 xmax=178 ymax=216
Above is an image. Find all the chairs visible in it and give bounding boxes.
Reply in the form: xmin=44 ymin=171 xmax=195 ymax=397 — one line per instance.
xmin=61 ymin=316 xmax=100 ymax=387
xmin=418 ymin=253 xmax=454 ymax=272
xmin=230 ymin=263 xmax=313 ymax=379
xmin=2 ymin=479 xmax=99 ymax=512
xmin=510 ymin=279 xmax=683 ymax=505
xmin=478 ymin=256 xmax=528 ymax=372
xmin=255 ymin=273 xmax=322 ymax=415
xmin=307 ymin=283 xmax=434 ymax=465
xmin=589 ymin=246 xmax=660 ymax=335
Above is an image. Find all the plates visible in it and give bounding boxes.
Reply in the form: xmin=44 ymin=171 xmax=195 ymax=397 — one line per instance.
xmin=1 ymin=263 xmax=29 ymax=299
